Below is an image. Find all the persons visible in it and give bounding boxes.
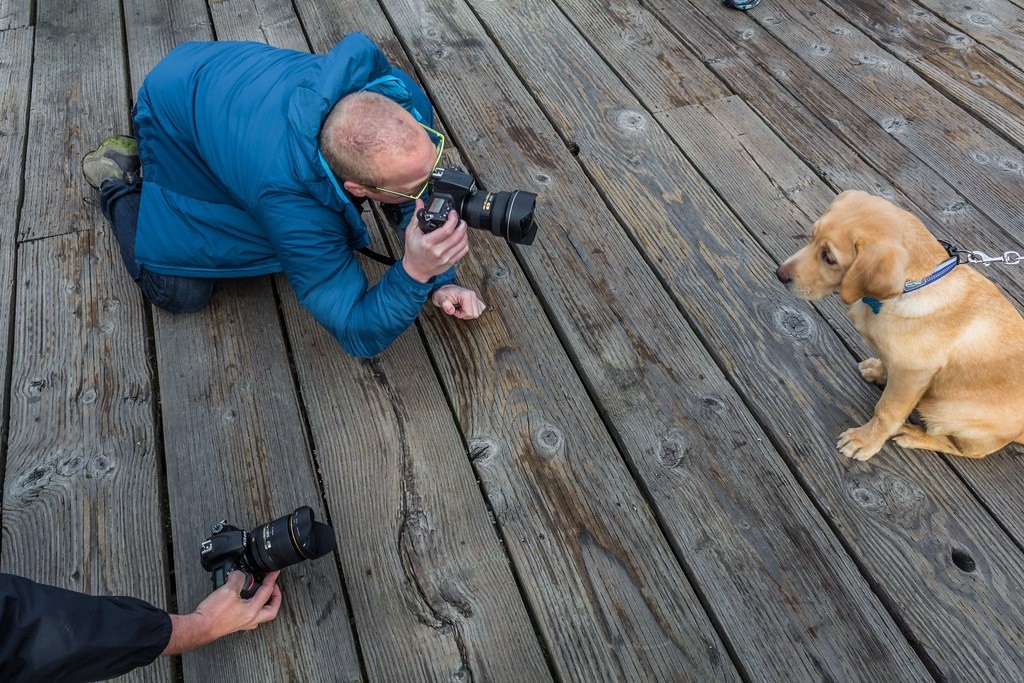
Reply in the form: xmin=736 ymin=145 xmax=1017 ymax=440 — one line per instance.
xmin=81 ymin=30 xmax=487 ymax=360
xmin=0 ymin=570 xmax=282 ymax=683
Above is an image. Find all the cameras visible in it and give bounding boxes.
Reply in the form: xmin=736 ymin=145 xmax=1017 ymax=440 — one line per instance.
xmin=200 ymin=505 xmax=338 ymax=606
xmin=417 ymin=166 xmax=538 ymax=246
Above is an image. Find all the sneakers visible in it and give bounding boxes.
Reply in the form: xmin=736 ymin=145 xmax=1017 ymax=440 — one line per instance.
xmin=82 ymin=134 xmax=141 ymax=190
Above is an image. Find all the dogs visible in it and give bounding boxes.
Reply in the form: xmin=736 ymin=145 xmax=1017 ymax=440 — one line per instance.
xmin=776 ymin=189 xmax=1024 ymax=462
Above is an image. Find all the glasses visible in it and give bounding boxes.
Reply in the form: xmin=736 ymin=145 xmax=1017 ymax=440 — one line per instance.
xmin=357 ymin=120 xmax=445 ymax=200
